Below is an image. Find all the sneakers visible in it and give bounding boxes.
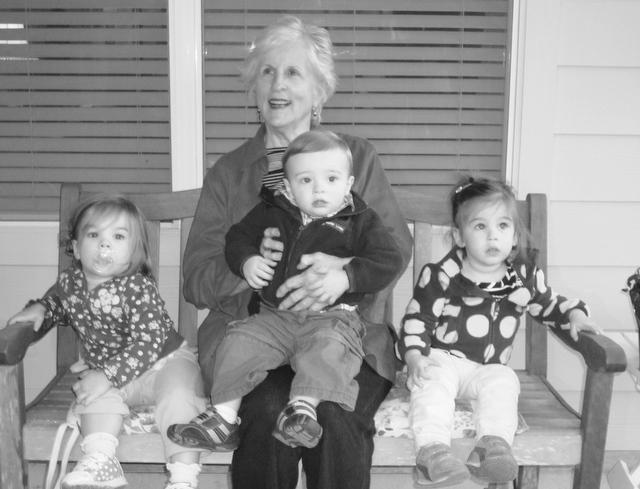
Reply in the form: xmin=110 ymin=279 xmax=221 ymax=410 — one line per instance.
xmin=416 ymin=442 xmax=471 ymax=489
xmin=61 ymin=449 xmax=129 ymax=489
xmin=465 ymin=435 xmax=519 ymax=484
xmin=167 ymin=407 xmax=242 ymax=452
xmin=271 ymin=400 xmax=323 ymax=449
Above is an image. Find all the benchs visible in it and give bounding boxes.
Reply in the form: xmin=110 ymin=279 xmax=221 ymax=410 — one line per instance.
xmin=0 ymin=182 xmax=626 ymax=489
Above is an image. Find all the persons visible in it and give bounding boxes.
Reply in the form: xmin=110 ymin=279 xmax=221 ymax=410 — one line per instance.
xmin=182 ymin=15 xmax=412 ymax=489
xmin=166 ymin=129 xmax=403 ymax=452
xmin=395 ymin=176 xmax=601 ymax=489
xmin=7 ymin=196 xmax=203 ymax=489
